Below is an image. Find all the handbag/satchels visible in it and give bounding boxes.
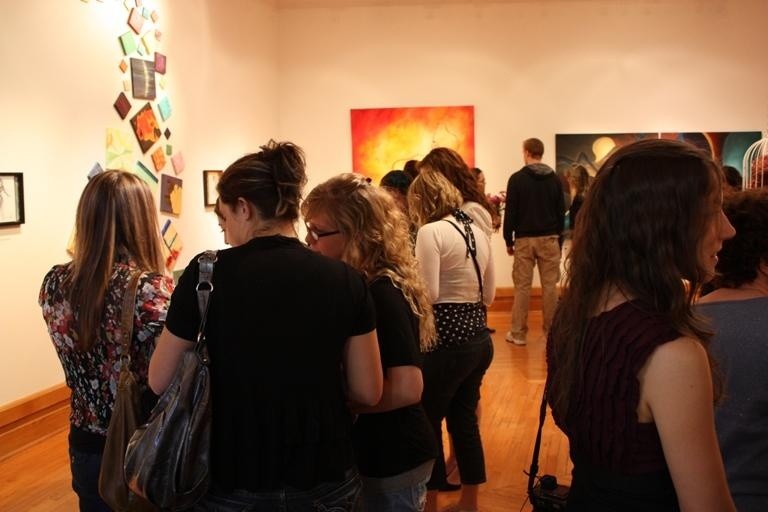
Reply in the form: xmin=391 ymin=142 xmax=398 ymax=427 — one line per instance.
xmin=122 ymin=243 xmax=220 ymax=505
xmin=98 ymin=270 xmax=158 ymax=510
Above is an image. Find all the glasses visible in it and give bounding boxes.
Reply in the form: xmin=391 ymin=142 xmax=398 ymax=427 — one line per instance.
xmin=304 ymin=220 xmax=341 ymax=243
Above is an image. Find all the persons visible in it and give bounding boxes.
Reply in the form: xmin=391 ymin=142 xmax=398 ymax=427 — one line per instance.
xmin=38 ymin=168 xmax=198 ymax=510
xmin=213 ymin=197 xmax=228 ymax=244
xmin=380 ymin=147 xmax=507 ymax=511
xmin=690 ymin=186 xmax=768 ymax=511
xmin=149 ymin=137 xmax=382 ymax=511
xmin=301 ymin=172 xmax=441 ymax=512
xmin=543 ymin=138 xmax=737 ymax=512
xmin=503 ymin=137 xmax=567 ymax=346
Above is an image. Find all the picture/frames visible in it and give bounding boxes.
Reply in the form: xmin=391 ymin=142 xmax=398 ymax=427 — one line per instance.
xmin=204 ymin=170 xmax=223 ymax=206
xmin=0 ymin=172 xmax=24 ymax=226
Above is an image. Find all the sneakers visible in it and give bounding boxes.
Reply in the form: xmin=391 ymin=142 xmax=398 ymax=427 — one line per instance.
xmin=505 ymin=330 xmax=527 ymax=346
